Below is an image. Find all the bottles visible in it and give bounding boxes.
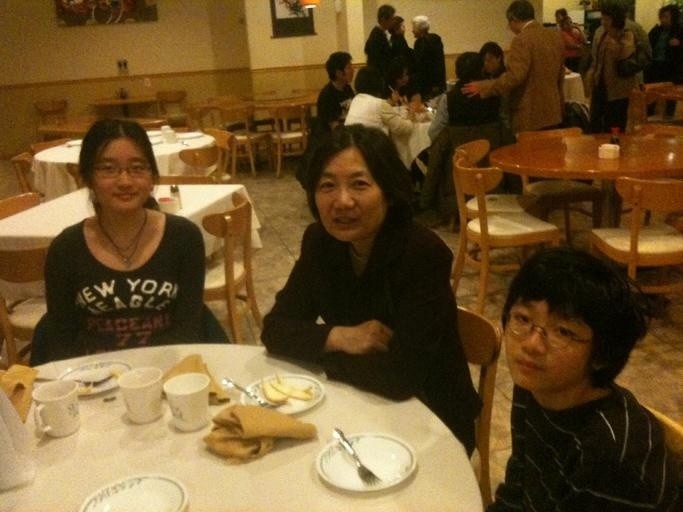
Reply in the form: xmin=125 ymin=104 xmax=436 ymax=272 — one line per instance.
xmin=169 ymin=185 xmax=182 ymax=209
xmin=610 ymin=127 xmax=620 ymax=144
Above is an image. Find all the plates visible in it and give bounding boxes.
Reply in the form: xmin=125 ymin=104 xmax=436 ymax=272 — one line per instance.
xmin=178 ymin=132 xmax=203 ymax=138
xmin=78 ymin=473 xmax=189 ymax=512
xmin=57 ymin=359 xmax=133 ymax=396
xmin=315 ymin=431 xmax=416 ymax=492
xmin=240 ymin=373 xmax=325 ymax=415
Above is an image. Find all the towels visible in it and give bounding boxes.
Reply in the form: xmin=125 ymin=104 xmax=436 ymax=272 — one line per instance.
xmin=203 ymin=404 xmax=318 ymax=465
xmin=2 ymin=392 xmax=32 ymax=493
xmin=162 ymin=352 xmax=230 ymax=407
xmin=2 ymin=364 xmax=37 ymax=424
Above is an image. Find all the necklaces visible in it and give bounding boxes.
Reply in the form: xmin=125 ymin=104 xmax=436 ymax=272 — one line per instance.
xmin=99 ymin=209 xmax=147 ymax=268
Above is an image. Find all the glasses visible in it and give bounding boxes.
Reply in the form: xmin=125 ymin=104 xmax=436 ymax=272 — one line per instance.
xmin=88 ymin=158 xmax=153 ymax=180
xmin=505 ymin=310 xmax=595 ymax=351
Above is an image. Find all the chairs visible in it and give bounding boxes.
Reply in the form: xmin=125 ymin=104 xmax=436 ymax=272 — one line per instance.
xmin=0 ymin=192 xmax=40 ymax=220
xmin=638 ymin=403 xmax=682 ymax=458
xmin=635 ymin=124 xmax=683 ymax=137
xmin=516 ymin=127 xmax=602 ymax=242
xmin=158 ymin=89 xmax=187 ymax=113
xmin=34 ymin=97 xmax=66 ymax=123
xmin=641 ymin=81 xmax=682 ymax=123
xmin=31 ymin=138 xmax=68 ymax=154
xmin=1 ymin=245 xmax=48 ymax=370
xmin=156 ymin=175 xmax=219 ymax=185
xmin=206 ymin=129 xmax=233 ymax=177
xmin=452 ymin=158 xmax=558 ymax=314
xmin=222 ymin=107 xmax=271 ymax=178
xmin=455 ymin=306 xmax=503 ymax=512
xmin=592 ymin=176 xmax=682 ymax=295
xmin=179 ymin=144 xmax=225 ymax=184
xmin=271 ymin=103 xmax=309 ymax=178
xmin=454 ymin=139 xmax=546 ymax=263
xmin=203 ymin=192 xmax=264 ymax=344
xmin=11 ymin=151 xmax=32 ymax=193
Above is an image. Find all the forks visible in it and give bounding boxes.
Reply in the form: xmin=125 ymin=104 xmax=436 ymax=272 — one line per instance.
xmin=331 ymin=428 xmax=382 ymax=487
xmin=224 ymin=375 xmax=276 ymax=410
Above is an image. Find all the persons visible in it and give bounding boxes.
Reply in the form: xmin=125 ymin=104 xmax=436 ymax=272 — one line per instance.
xmin=343 ymin=65 xmax=422 ymax=143
xmin=458 ymin=0 xmax=566 ymax=193
xmin=428 ymin=51 xmax=500 ymax=143
xmin=261 ymin=123 xmax=484 ymax=461
xmin=588 ymin=5 xmax=645 ymax=133
xmin=591 ymin=0 xmax=653 ymax=74
xmin=387 ymin=15 xmax=414 ymax=95
xmin=407 ymin=14 xmax=447 ymax=102
xmin=580 ymin=0 xmax=594 ymax=42
xmin=556 ymin=9 xmax=589 ymax=73
xmin=479 ymin=41 xmax=512 ymax=135
xmin=579 ymin=35 xmax=594 ymax=97
xmin=644 ymin=4 xmax=682 ymax=116
xmin=43 ymin=117 xmax=207 ymax=362
xmin=316 ymin=52 xmax=356 ymax=131
xmin=560 ymin=16 xmax=586 ymax=58
xmin=364 ymin=5 xmax=395 ymax=97
xmin=384 ymin=54 xmax=412 ymax=105
xmin=485 ymin=244 xmax=682 ymax=510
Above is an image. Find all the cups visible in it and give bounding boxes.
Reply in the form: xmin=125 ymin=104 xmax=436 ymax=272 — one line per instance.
xmin=30 ymin=379 xmax=81 ymax=438
xmin=115 ymin=366 xmax=163 ymax=424
xmin=158 ymin=198 xmax=176 ymax=213
xmin=160 ymin=124 xmax=177 ymax=145
xmin=161 ymin=372 xmax=211 ymax=432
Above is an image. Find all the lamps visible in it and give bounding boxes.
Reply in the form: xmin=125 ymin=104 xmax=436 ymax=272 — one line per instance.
xmin=300 ymin=0 xmax=321 ymax=8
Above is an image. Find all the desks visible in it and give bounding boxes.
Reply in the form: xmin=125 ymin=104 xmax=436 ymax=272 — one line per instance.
xmin=33 ymin=130 xmax=222 ymax=198
xmin=387 ymin=101 xmax=441 ymax=175
xmin=38 ymin=117 xmax=167 ymax=139
xmin=2 ymin=341 xmax=485 ymax=512
xmin=563 ymin=71 xmax=585 ymax=106
xmin=490 ymin=118 xmax=683 ymax=227
xmin=0 ymin=184 xmax=262 ymax=300
xmin=90 ymin=95 xmax=157 ymax=117
xmin=238 ymin=88 xmax=320 ymax=164
